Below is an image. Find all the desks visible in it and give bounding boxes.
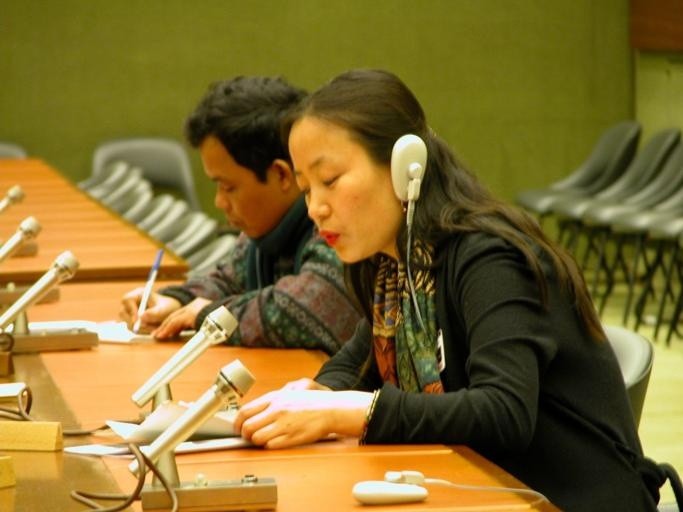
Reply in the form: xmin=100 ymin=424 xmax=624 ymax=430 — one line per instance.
xmin=0 ymin=159 xmax=190 ymax=288
xmin=0 ymin=280 xmax=564 ymax=512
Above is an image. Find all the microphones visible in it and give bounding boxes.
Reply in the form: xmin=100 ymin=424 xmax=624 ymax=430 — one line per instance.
xmin=0 ymin=251 xmax=99 ymax=353
xmin=128 ymin=359 xmax=277 ymax=510
xmin=0 ymin=216 xmax=42 ymax=262
xmin=0 ymin=184 xmax=26 ymax=214
xmin=132 ymin=305 xmax=240 ymax=413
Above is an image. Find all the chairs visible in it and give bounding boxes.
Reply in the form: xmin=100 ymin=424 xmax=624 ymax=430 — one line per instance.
xmin=511 ymin=120 xmax=683 ymax=348
xmin=600 ymin=322 xmax=655 ymax=438
xmin=74 ymin=138 xmax=238 ymax=278
xmin=0 ymin=143 xmax=26 ymax=159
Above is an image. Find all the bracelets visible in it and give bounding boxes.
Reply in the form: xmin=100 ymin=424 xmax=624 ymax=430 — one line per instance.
xmin=358 ymin=388 xmax=379 ymax=445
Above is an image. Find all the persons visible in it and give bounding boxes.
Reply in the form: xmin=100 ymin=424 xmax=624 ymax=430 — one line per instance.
xmin=119 ymin=77 xmax=363 ymax=356
xmin=228 ymin=68 xmax=662 ymax=512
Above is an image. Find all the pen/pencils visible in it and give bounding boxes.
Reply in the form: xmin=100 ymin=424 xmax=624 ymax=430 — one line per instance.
xmin=132 ymin=249 xmax=163 ymax=335
